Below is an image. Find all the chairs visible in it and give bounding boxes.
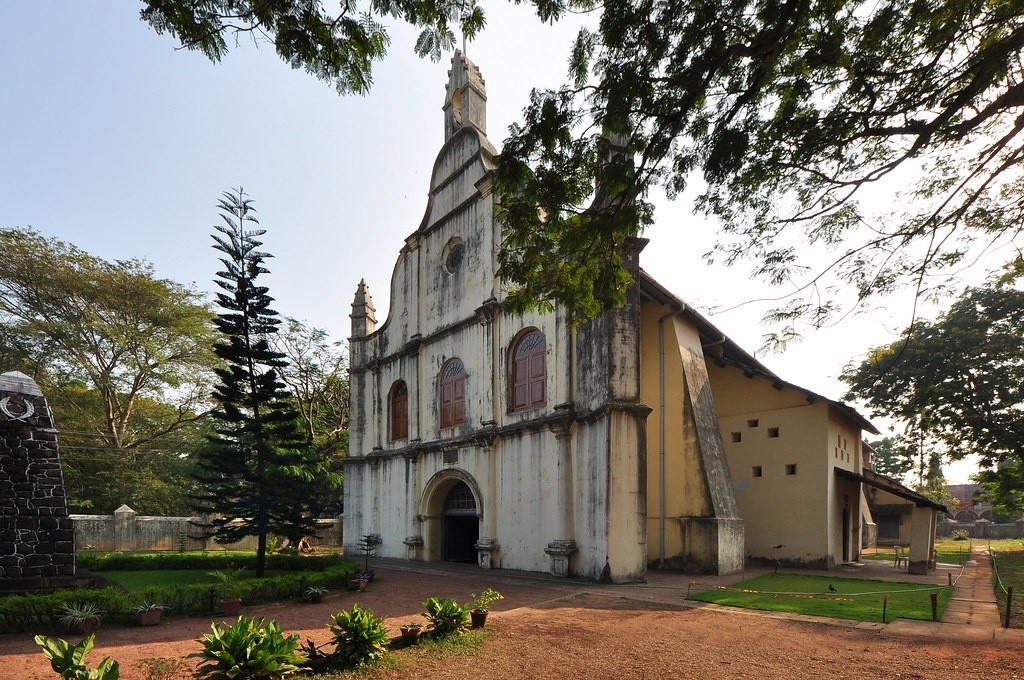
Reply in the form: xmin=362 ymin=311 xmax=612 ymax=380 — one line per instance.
xmin=930 ymin=548 xmax=938 ymax=572
xmin=893 ymin=545 xmax=909 ymax=569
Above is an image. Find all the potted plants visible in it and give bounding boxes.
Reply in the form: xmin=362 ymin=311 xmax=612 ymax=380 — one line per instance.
xmin=206 ymin=566 xmax=247 ymax=617
xmin=350 ymin=573 xmax=371 ymax=591
xmin=400 ymin=624 xmax=423 ymax=645
xmin=56 ymin=599 xmax=107 ymax=633
xmin=132 ymin=600 xmax=172 ymax=627
xmin=469 ymin=588 xmax=504 ymax=628
xmin=304 ymin=586 xmax=331 ymax=603
